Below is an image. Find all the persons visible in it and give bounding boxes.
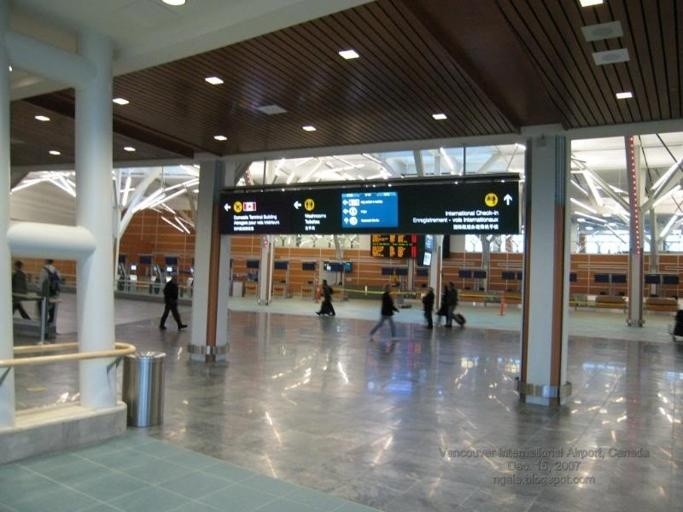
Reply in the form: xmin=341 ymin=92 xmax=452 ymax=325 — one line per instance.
xmin=13 ymin=258 xmax=682 ymax=341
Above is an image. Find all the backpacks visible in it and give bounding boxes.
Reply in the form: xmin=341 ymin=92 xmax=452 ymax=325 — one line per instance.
xmin=41 ymin=265 xmax=61 ymax=298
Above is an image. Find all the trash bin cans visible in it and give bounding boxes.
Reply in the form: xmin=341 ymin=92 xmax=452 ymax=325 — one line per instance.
xmin=122 ymin=350 xmax=166 ymax=427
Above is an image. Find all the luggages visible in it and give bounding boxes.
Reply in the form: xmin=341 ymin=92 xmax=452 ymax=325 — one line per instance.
xmin=451 ymin=312 xmax=465 ymax=325
